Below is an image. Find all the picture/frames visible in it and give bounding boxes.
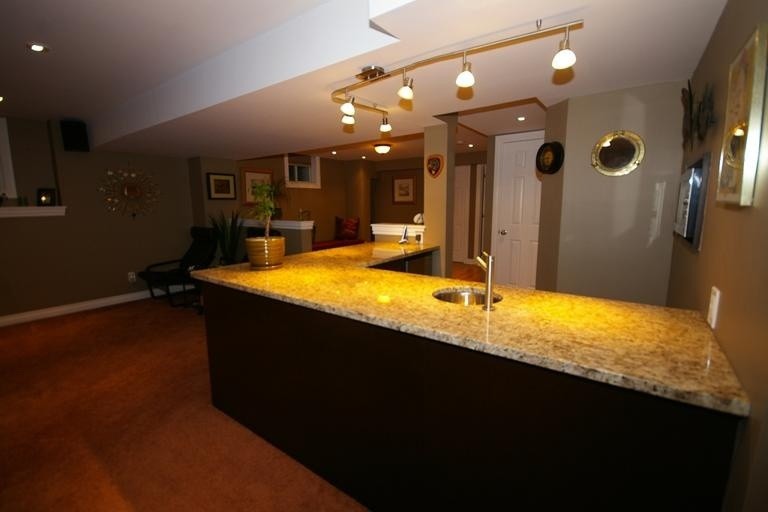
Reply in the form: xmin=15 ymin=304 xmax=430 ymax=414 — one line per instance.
xmin=715 ymin=23 xmax=767 ymax=207
xmin=391 ymin=176 xmax=417 ymax=206
xmin=241 ymin=167 xmax=273 ymax=209
xmin=206 ymin=172 xmax=237 ymax=200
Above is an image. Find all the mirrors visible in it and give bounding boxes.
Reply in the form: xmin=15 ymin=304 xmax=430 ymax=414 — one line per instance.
xmin=590 ymin=130 xmax=645 ymax=177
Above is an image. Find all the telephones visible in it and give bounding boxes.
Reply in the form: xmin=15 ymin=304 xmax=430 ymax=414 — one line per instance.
xmin=398 ymin=226 xmax=409 ymax=244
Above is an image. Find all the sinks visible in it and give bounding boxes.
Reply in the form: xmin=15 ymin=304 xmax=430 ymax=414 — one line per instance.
xmin=430 ymin=282 xmax=505 ymax=310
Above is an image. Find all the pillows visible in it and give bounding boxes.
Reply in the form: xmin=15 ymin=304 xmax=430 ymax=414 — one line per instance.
xmin=335 ymin=216 xmax=360 ymax=239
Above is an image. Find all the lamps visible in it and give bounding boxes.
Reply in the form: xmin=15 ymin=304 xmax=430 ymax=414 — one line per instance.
xmin=331 ymin=17 xmax=584 ymax=133
xmin=372 ymin=144 xmax=392 ymax=153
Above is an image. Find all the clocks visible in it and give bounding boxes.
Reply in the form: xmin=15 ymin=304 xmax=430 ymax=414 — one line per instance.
xmin=536 ymin=141 xmax=564 ymax=174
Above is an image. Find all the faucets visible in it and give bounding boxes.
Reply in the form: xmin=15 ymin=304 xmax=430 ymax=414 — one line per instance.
xmin=476 ymin=249 xmax=497 ymax=311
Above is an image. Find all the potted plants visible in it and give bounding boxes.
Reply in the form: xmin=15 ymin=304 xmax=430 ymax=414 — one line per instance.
xmin=247 ymin=176 xmax=289 ymax=270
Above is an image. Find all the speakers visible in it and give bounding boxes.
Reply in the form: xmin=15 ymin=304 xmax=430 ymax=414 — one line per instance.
xmin=61 ymin=119 xmax=90 ymax=152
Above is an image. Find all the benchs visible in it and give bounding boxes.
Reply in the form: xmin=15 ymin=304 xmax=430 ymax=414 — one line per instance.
xmin=313 ymin=239 xmax=367 ymax=251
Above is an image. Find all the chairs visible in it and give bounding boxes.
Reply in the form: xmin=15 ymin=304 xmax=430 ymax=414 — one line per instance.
xmin=137 ymin=226 xmax=219 ymax=307
